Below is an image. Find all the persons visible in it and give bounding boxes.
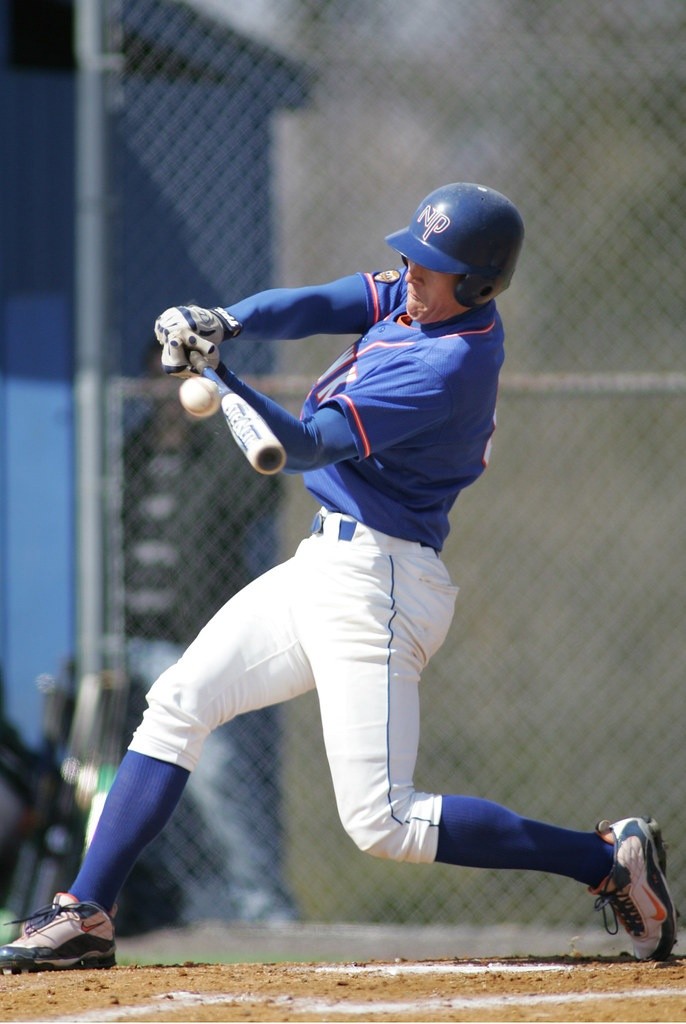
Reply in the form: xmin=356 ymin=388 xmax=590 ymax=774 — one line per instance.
xmin=2 ymin=181 xmax=679 ymax=971
xmin=113 ymin=345 xmax=305 ymax=926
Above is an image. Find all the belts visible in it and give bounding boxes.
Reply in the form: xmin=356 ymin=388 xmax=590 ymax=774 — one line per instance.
xmin=311 ymin=513 xmax=357 ymax=542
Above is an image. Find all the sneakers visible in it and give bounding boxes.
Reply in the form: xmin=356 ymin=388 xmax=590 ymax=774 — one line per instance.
xmin=587 ymin=817 xmax=677 ymax=959
xmin=1 ymin=894 xmax=117 ymax=973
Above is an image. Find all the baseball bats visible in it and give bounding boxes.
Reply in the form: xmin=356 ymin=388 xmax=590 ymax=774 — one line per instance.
xmin=178 ymin=341 xmax=290 ymax=477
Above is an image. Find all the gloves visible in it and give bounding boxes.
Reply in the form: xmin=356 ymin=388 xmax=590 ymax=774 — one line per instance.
xmin=160 ymin=328 xmax=220 ymax=379
xmin=153 ymin=304 xmax=240 ymax=347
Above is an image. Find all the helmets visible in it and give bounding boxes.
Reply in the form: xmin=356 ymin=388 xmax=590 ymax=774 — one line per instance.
xmin=384 ymin=181 xmax=525 ymax=307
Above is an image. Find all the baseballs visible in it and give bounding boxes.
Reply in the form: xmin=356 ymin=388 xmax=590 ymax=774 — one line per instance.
xmin=176 ymin=373 xmax=226 ymax=421
